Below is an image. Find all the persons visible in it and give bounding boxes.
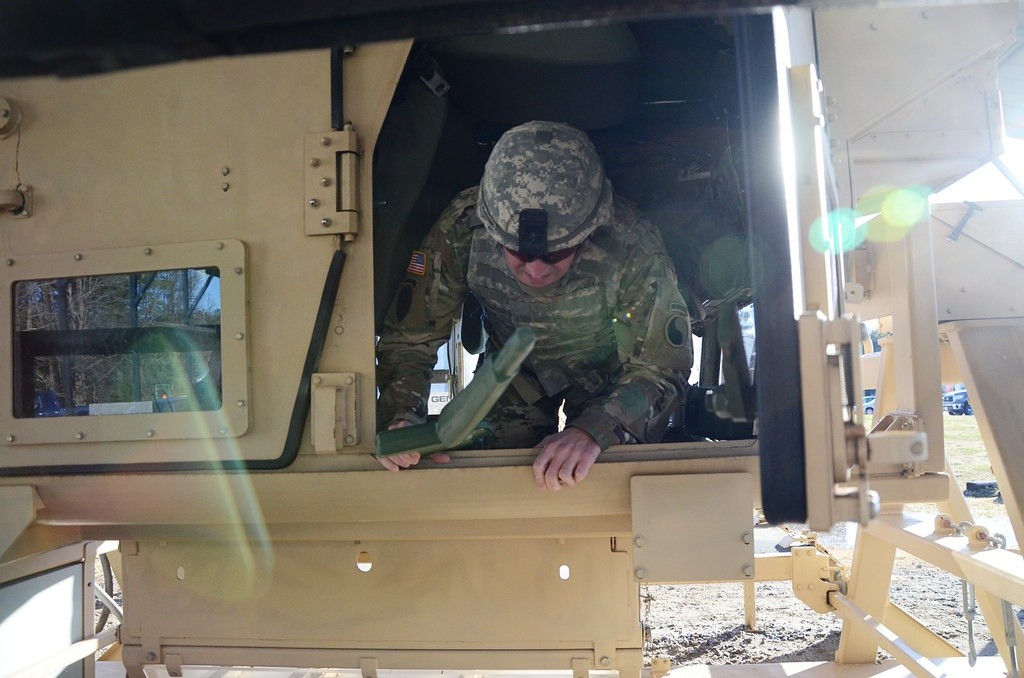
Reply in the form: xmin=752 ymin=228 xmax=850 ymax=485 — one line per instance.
xmin=374 ymin=121 xmax=693 ymax=490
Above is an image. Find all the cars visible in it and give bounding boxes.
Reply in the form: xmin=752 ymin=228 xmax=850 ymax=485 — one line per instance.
xmin=852 ymin=395 xmax=875 ymax=415
xmin=943 ymin=389 xmax=972 ymax=415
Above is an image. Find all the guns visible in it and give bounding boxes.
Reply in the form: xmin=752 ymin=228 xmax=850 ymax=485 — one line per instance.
xmin=375 ymin=326 xmax=537 ymax=459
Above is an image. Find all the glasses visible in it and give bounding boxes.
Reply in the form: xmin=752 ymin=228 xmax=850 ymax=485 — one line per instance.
xmin=503 ymin=245 xmax=579 ymax=264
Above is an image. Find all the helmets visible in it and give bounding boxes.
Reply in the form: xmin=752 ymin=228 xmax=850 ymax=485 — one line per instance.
xmin=477 ymin=121 xmax=613 ymax=253
